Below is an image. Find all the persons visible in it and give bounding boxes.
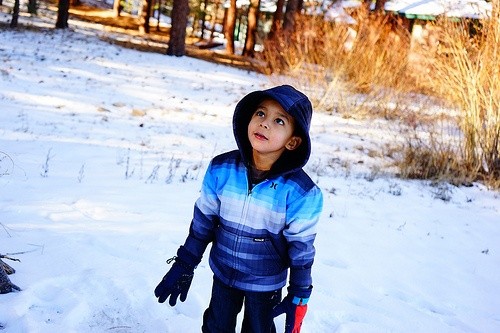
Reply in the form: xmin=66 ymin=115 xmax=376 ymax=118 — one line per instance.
xmin=154 ymin=85 xmax=323 ymax=333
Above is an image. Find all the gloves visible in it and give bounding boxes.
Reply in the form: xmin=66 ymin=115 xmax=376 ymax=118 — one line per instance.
xmin=272 ymin=285 xmax=314 ymax=333
xmin=154 ymin=245 xmax=203 ymax=307
